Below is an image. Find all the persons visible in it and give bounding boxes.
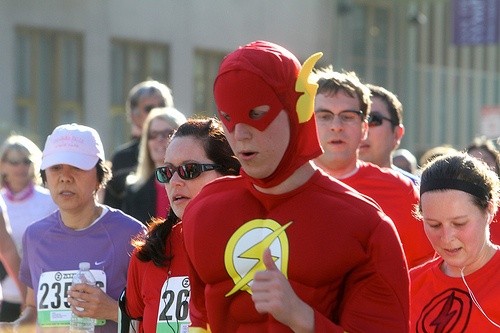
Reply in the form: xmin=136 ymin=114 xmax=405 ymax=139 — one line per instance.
xmin=0 ymin=40 xmax=500 ymax=333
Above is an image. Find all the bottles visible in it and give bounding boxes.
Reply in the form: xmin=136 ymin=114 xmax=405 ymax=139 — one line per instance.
xmin=67 ymin=261 xmax=96 ymax=333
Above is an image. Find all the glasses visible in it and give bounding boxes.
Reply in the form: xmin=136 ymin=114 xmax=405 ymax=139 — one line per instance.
xmin=314 ymin=109 xmax=364 ymax=123
xmin=4 ymin=158 xmax=31 ymax=165
xmin=368 ymin=115 xmax=399 ymax=128
xmin=154 ymin=163 xmax=222 ymax=183
xmin=137 ymin=105 xmax=153 ymax=114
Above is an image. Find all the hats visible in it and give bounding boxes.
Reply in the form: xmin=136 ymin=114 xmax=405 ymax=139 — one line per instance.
xmin=41 ymin=123 xmax=105 ymax=171
